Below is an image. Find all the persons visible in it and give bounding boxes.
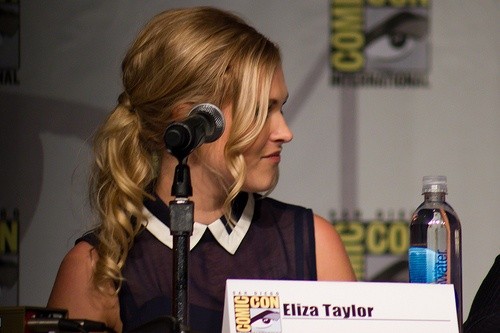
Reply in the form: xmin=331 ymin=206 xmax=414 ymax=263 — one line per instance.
xmin=47 ymin=6 xmax=357 ymax=333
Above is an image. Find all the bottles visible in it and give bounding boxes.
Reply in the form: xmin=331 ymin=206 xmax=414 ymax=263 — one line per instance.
xmin=409 ymin=175 xmax=463 ymax=333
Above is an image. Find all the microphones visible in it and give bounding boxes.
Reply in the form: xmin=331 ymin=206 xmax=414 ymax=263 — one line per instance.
xmin=163 ymin=104 xmax=226 ymax=155
